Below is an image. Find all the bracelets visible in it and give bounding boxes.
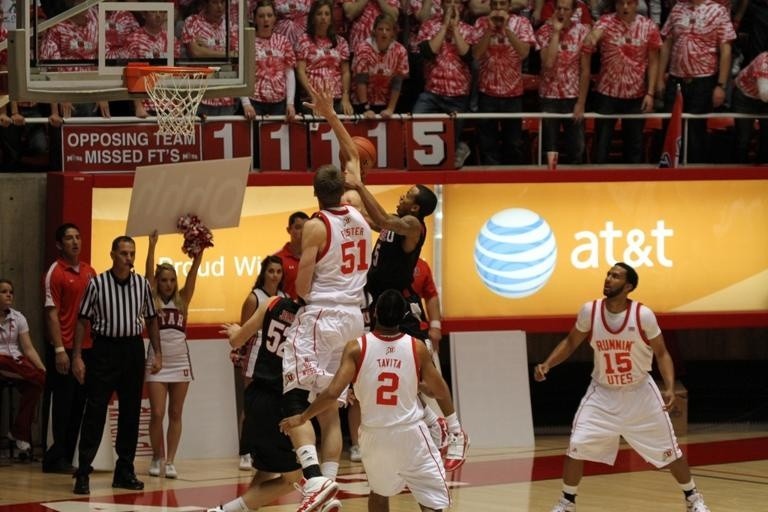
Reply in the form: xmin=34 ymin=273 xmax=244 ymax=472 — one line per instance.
xmin=54 ymin=346 xmax=64 ymax=353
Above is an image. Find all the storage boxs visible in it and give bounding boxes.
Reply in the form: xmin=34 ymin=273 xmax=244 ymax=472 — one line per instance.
xmin=652 ymin=376 xmax=688 ymax=439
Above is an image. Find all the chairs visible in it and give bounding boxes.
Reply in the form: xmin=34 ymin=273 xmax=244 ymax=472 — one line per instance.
xmin=470 ymin=71 xmax=762 ymax=163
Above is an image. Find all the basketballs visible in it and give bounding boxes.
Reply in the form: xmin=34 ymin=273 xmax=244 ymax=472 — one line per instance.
xmin=339 ymin=136 xmax=376 ymax=171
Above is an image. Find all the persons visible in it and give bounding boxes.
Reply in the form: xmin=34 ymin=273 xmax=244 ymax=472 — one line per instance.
xmin=40 ymin=224 xmax=98 ymax=474
xmin=534 ymin=261 xmax=712 ymax=512
xmin=71 ymin=236 xmax=163 ymax=495
xmin=207 ymin=212 xmax=449 ymax=512
xmin=143 ymin=230 xmax=204 ymax=478
xmin=280 ymin=77 xmax=374 ymax=512
xmin=340 ymin=170 xmax=473 ymax=470
xmin=1 ymin=279 xmax=46 ymax=462
xmin=0 ymin=0 xmax=768 ymax=169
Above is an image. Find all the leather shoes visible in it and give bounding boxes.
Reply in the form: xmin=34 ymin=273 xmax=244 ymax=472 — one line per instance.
xmin=43 ymin=462 xmax=77 ymax=474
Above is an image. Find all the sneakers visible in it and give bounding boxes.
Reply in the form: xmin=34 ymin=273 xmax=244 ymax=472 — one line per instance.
xmin=149 ymin=459 xmax=177 ymax=478
xmin=73 ymin=473 xmax=89 ymax=494
xmin=239 ymin=455 xmax=252 ymax=470
xmin=429 ymin=417 xmax=470 ymax=471
xmin=292 ymin=476 xmax=343 ymax=512
xmin=7 ymin=432 xmax=31 ymax=450
xmin=686 ymin=490 xmax=711 ymax=512
xmin=349 ymin=446 xmax=361 ymax=462
xmin=113 ymin=472 xmax=144 ymax=490
xmin=551 ymin=491 xmax=576 ymax=512
